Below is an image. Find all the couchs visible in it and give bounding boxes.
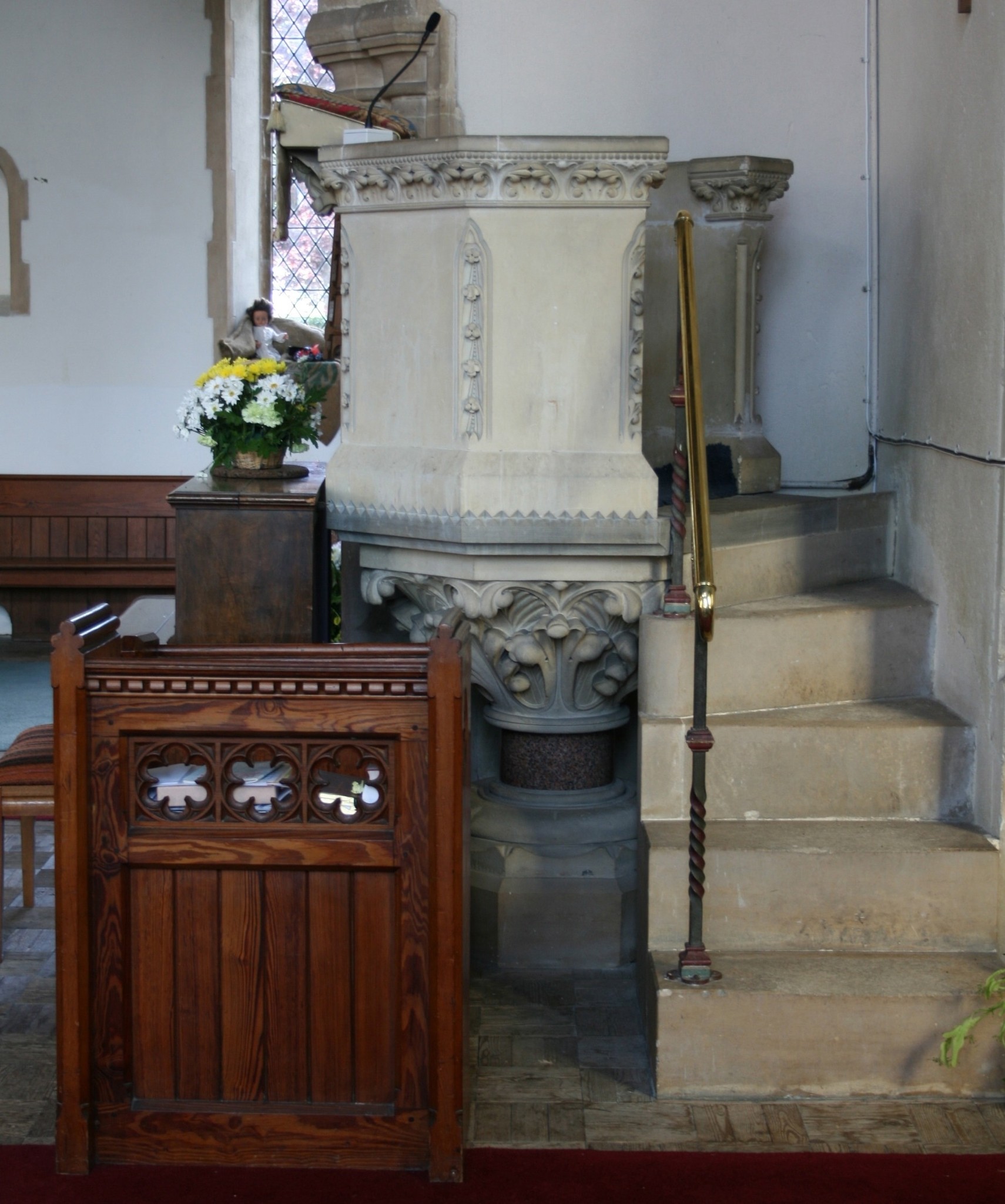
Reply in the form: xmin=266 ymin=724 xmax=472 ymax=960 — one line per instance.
xmin=217 ymin=314 xmax=329 ymax=363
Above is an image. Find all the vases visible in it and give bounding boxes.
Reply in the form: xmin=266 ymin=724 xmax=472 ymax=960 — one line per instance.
xmin=230 ymin=448 xmax=286 ymax=470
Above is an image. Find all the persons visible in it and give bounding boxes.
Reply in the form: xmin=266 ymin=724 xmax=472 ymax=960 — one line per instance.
xmin=246 ymin=297 xmax=289 ymax=361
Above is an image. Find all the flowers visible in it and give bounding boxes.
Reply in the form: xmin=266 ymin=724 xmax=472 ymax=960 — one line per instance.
xmin=169 ymin=356 xmax=333 ymax=473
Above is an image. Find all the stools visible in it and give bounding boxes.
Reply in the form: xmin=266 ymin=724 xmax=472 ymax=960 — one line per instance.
xmin=0 ymin=721 xmax=55 ymax=910
